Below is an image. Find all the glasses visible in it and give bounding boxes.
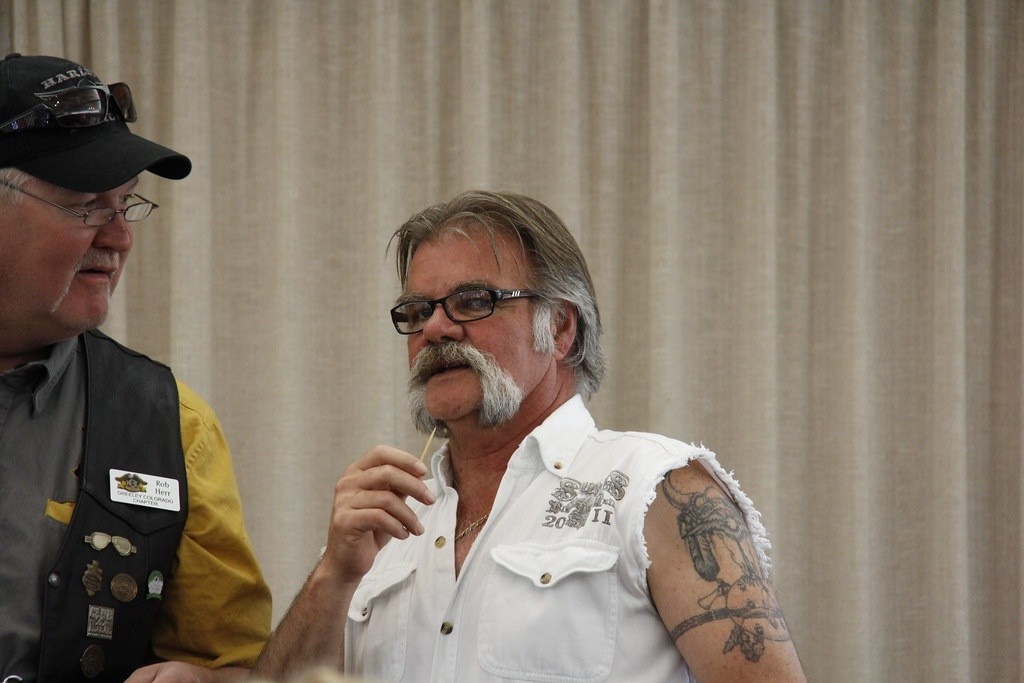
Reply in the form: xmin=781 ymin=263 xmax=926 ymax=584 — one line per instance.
xmin=390 ymin=289 xmax=547 ymax=335
xmin=0 ymin=82 xmax=137 ymax=141
xmin=1 ymin=180 xmax=159 ymax=226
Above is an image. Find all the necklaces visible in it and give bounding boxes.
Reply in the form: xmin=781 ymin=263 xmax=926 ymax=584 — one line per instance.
xmin=455 ymin=514 xmax=489 ymax=541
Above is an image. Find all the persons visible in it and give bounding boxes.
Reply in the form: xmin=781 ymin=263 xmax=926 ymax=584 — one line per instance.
xmin=248 ymin=190 xmax=807 ymax=683
xmin=0 ymin=51 xmax=272 ymax=683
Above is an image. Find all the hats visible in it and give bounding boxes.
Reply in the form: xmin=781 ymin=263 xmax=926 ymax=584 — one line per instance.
xmin=0 ymin=53 xmax=191 ymax=193
